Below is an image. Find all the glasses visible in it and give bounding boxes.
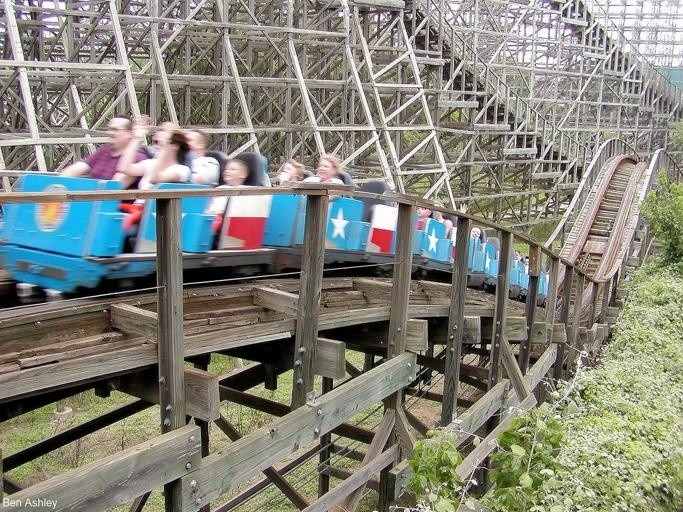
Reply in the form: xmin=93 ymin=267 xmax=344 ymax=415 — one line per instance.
xmin=106 ymin=127 xmax=131 ymax=132
xmin=153 ymin=139 xmax=165 ymax=145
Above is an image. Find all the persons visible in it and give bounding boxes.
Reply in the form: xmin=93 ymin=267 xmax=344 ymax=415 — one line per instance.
xmin=61 ymin=115 xmax=534 ymax=275
